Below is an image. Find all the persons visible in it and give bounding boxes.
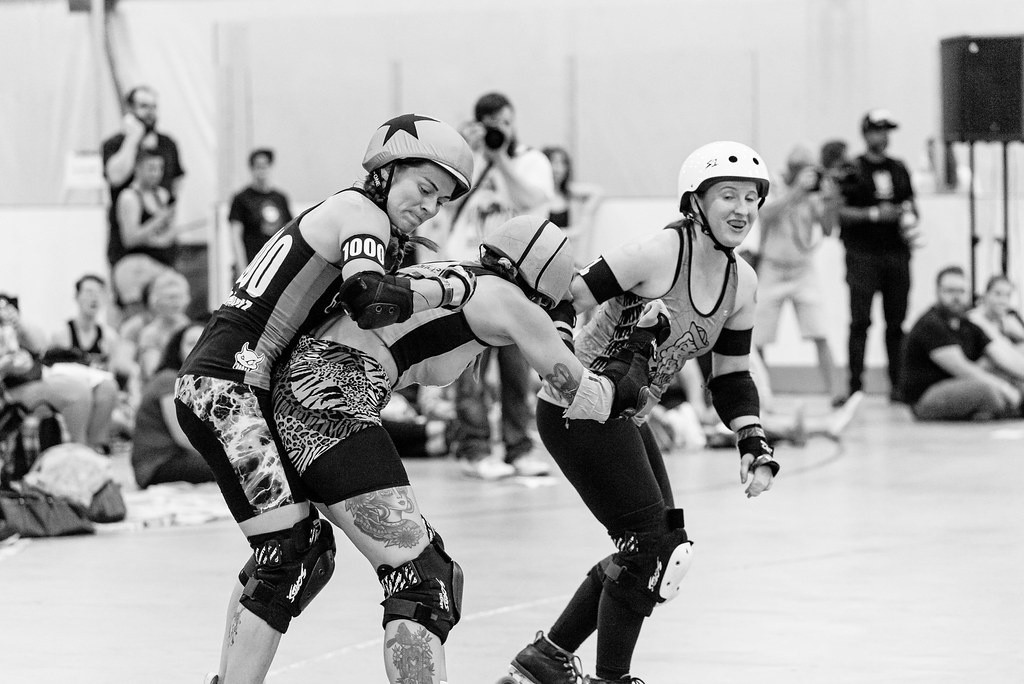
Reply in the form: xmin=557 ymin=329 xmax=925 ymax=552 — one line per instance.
xmin=0 ymin=86 xmax=1024 ymax=684
xmin=174 ymin=112 xmax=478 ymax=684
xmin=506 ymin=139 xmax=780 ymax=684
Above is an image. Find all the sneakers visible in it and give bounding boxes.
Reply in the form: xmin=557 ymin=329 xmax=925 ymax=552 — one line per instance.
xmin=508 ymin=630 xmax=583 ymax=684
xmin=583 ymin=673 xmax=645 ymax=684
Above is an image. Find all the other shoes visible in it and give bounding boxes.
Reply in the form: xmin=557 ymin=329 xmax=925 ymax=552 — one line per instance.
xmin=513 ymin=450 xmax=549 ymax=474
xmin=463 ymin=456 xmax=515 ymax=479
xmin=828 ymin=391 xmax=861 ymax=436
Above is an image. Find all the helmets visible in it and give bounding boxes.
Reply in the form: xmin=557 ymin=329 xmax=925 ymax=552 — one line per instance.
xmin=483 ymin=215 xmax=575 ymax=310
xmin=362 ymin=113 xmax=473 ymax=202
xmin=678 ymin=141 xmax=770 ymax=213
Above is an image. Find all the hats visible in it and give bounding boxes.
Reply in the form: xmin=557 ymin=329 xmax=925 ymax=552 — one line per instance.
xmin=862 ymin=109 xmax=898 ymax=132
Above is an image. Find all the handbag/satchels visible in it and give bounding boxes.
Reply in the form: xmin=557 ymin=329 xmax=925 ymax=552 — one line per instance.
xmin=0 ymin=479 xmax=95 ymax=535
